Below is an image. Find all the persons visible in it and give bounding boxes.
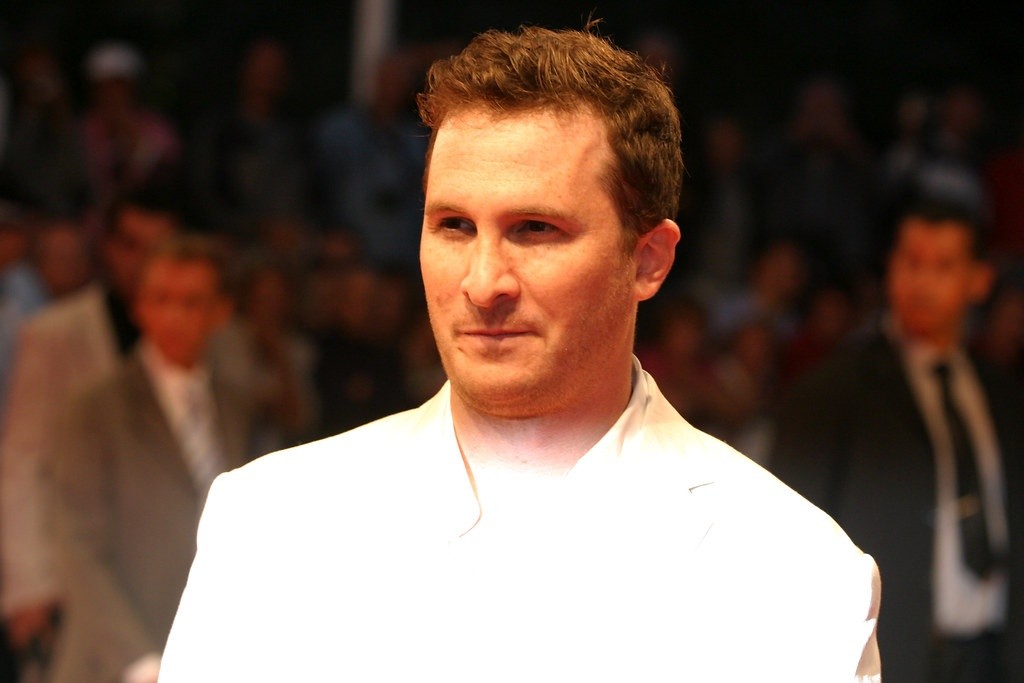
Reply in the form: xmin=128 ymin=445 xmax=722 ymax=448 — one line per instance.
xmin=0 ymin=1 xmax=447 ymax=682
xmin=792 ymin=197 xmax=1023 ymax=683
xmin=155 ymin=29 xmax=880 ymax=682
xmin=635 ymin=34 xmax=1024 ymax=458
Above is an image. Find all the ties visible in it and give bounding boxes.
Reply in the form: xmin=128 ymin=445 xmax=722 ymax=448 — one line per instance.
xmin=932 ymin=359 xmax=990 ymax=570
